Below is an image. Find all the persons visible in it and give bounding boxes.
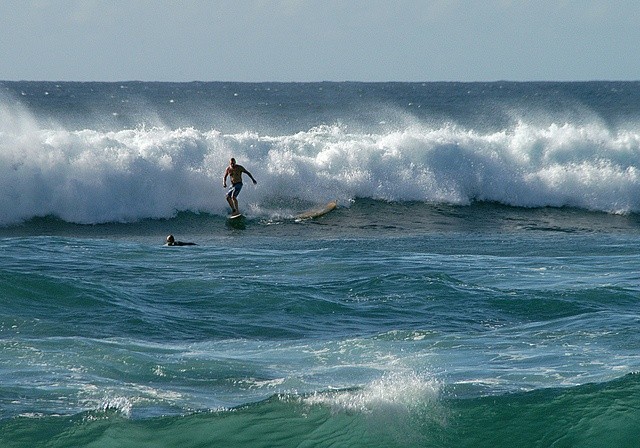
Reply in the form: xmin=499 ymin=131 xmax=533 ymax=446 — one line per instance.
xmin=223 ymin=157 xmax=257 ymax=216
xmin=163 ymin=234 xmax=199 ymax=247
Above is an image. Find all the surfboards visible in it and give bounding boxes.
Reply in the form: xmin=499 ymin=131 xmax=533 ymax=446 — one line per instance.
xmin=225 ymin=213 xmax=247 ymax=231
